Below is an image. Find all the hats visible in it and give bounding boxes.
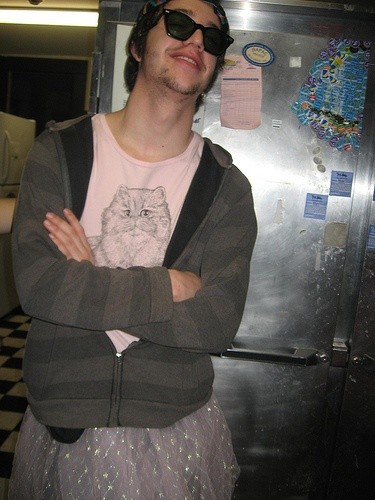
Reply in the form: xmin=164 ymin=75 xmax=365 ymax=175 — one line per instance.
xmin=124 ymin=0 xmax=230 ymax=92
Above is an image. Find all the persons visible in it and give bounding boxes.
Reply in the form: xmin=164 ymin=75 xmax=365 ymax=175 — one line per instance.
xmin=10 ymin=0 xmax=259 ymax=500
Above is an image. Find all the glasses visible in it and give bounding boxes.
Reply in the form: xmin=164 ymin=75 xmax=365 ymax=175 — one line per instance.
xmin=147 ymin=9 xmax=235 ymax=56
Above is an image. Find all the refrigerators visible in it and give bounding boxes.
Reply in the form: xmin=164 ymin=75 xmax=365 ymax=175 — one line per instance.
xmin=88 ymin=0 xmax=375 ymax=500
